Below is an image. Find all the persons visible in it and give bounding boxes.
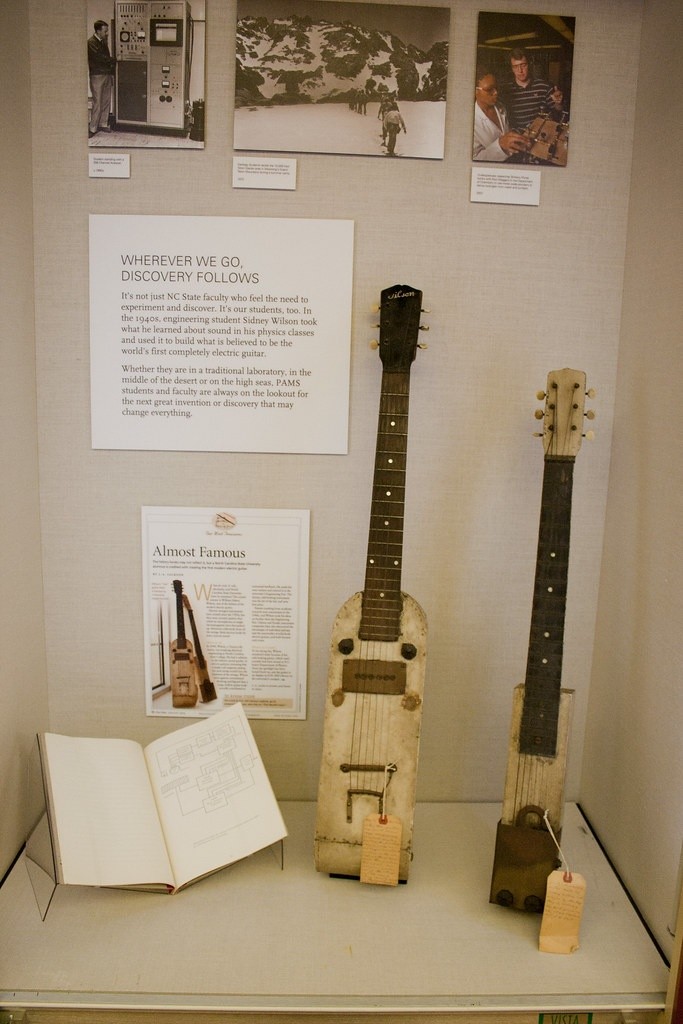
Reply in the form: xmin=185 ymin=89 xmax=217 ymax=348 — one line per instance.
xmin=377 ymin=91 xmax=407 ymax=156
xmin=347 ymin=87 xmax=370 ymax=116
xmin=473 ymin=67 xmax=532 ymax=162
xmin=86 ymin=19 xmax=123 ymax=139
xmin=494 ymin=46 xmax=562 ymax=162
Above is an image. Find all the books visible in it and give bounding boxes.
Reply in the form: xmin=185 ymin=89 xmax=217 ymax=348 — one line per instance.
xmin=35 ymin=700 xmax=290 ymax=896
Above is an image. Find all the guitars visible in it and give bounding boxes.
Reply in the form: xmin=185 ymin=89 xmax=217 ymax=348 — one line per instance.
xmin=488 ymin=366 xmax=597 ymax=916
xmin=168 ymin=579 xmax=199 ymax=710
xmin=313 ymin=284 xmax=430 ymax=889
xmin=182 ymin=594 xmax=218 ymax=705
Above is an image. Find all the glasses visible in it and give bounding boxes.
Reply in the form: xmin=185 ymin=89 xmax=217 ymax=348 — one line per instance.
xmin=477 ymin=86 xmax=500 ymax=93
